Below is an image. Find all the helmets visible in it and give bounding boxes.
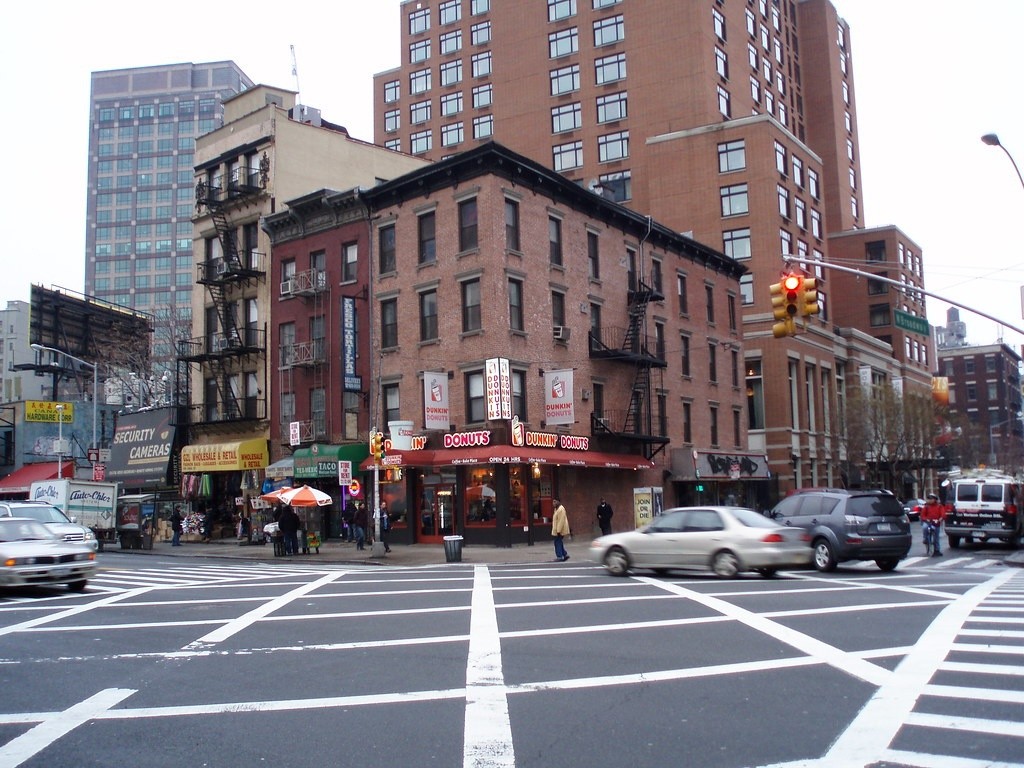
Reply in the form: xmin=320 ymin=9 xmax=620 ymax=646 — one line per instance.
xmin=927 ymin=494 xmax=937 ymax=500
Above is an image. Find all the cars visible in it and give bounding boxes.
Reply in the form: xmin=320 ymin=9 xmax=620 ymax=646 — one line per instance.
xmin=904 ymin=498 xmax=926 ymax=522
xmin=0 ymin=517 xmax=99 ymax=599
xmin=587 ymin=505 xmax=816 ymax=579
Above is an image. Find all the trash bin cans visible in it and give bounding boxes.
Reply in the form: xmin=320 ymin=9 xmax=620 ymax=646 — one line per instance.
xmin=443 ymin=535 xmax=464 ymax=563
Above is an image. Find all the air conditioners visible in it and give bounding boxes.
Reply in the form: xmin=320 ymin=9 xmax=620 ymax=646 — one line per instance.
xmin=280 ymin=280 xmax=295 ymax=294
xmin=219 ymin=338 xmax=230 ymax=350
xmin=217 ymin=262 xmax=229 ymax=276
xmin=594 ymin=417 xmax=610 ymax=429
xmin=553 ymin=326 xmax=570 ymax=341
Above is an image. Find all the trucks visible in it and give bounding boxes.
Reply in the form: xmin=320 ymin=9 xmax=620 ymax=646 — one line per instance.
xmin=25 ymin=479 xmax=119 ymax=552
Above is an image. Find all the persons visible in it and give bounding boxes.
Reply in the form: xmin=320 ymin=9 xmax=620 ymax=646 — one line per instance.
xmin=344 ymin=500 xmax=393 ymax=553
xmin=551 ymin=496 xmax=570 ymax=562
xmin=597 ymin=496 xmax=613 ymax=535
xmin=484 ymin=497 xmax=495 ymax=518
xmin=920 ymin=493 xmax=945 ymax=557
xmin=171 ymin=505 xmax=185 ymax=546
xmin=278 ymin=505 xmax=300 ymax=556
xmin=202 ymin=506 xmax=213 ymax=543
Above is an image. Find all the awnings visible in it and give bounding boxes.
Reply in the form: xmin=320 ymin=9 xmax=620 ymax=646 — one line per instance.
xmin=359 ymin=441 xmax=654 ymax=472
xmin=181 ymin=437 xmax=269 ymax=473
xmin=0 ymin=461 xmax=73 ymax=493
xmin=266 ymin=442 xmax=369 ymax=479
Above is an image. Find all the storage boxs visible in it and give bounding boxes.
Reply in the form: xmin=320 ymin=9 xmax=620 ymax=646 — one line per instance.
xmin=144 ymin=518 xmax=234 ymax=542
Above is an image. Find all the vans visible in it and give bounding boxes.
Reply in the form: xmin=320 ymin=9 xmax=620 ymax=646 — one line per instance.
xmin=944 ymin=475 xmax=1024 ymax=547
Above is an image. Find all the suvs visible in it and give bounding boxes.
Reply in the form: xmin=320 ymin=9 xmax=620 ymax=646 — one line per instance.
xmin=764 ymin=487 xmax=913 ymax=573
xmin=0 ymin=500 xmax=99 ymax=554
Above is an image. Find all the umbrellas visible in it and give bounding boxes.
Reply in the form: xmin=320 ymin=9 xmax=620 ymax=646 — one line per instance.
xmin=260 ymin=484 xmax=333 ymax=530
xmin=467 ymin=484 xmax=496 ymax=500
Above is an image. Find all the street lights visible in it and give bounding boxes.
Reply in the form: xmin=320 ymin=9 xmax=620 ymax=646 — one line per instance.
xmin=990 ymin=417 xmax=1024 ymax=453
xmin=30 ymin=341 xmax=99 ymax=482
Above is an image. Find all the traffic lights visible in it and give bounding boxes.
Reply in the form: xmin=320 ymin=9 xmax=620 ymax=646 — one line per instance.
xmin=785 ymin=276 xmax=805 ymax=318
xmin=375 ymin=435 xmax=386 ymax=463
xmin=804 ymin=278 xmax=820 ymax=315
xmin=769 ymin=282 xmax=796 ymax=337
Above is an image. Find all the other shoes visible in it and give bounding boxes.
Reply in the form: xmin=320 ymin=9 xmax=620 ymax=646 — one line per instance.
xmin=923 ymin=539 xmax=928 ymax=544
xmin=203 ymin=537 xmax=209 ymax=543
xmin=554 ymin=556 xmax=570 ymax=562
xmin=934 ymin=550 xmax=943 ymax=556
